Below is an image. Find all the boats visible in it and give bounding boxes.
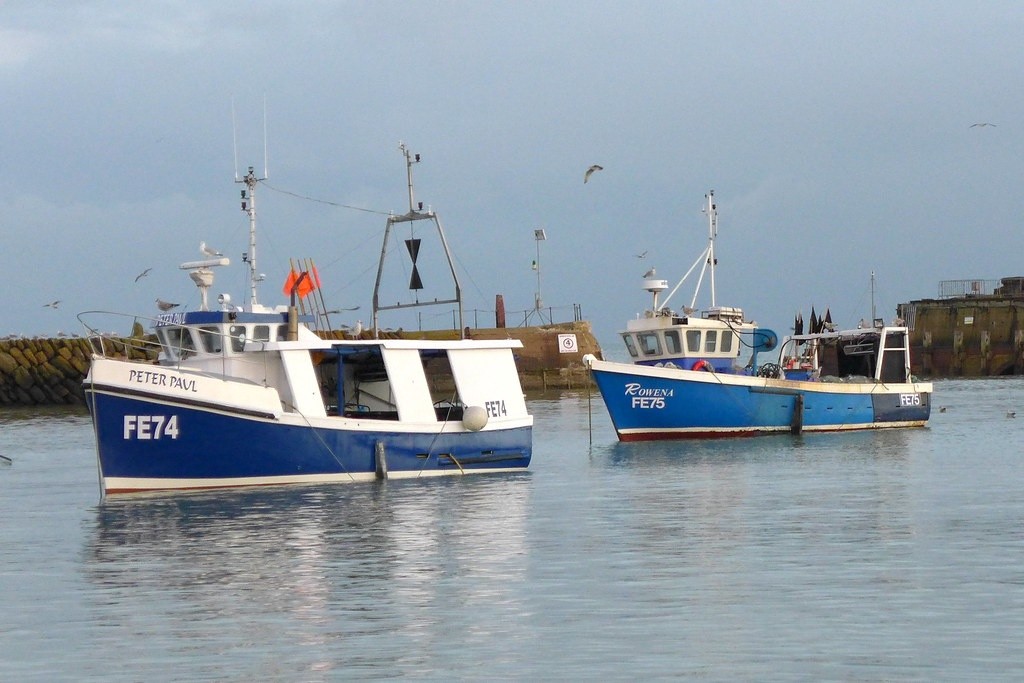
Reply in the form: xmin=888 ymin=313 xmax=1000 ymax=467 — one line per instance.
xmin=75 ymin=141 xmax=533 ymax=501
xmin=585 ymin=190 xmax=933 ymax=442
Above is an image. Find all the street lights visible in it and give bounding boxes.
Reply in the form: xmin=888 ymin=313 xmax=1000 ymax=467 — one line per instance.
xmin=534 ymin=229 xmax=552 ymax=328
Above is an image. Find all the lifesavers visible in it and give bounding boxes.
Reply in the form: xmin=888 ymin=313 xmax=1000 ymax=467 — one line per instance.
xmin=692 ymin=361 xmax=715 ymax=372
